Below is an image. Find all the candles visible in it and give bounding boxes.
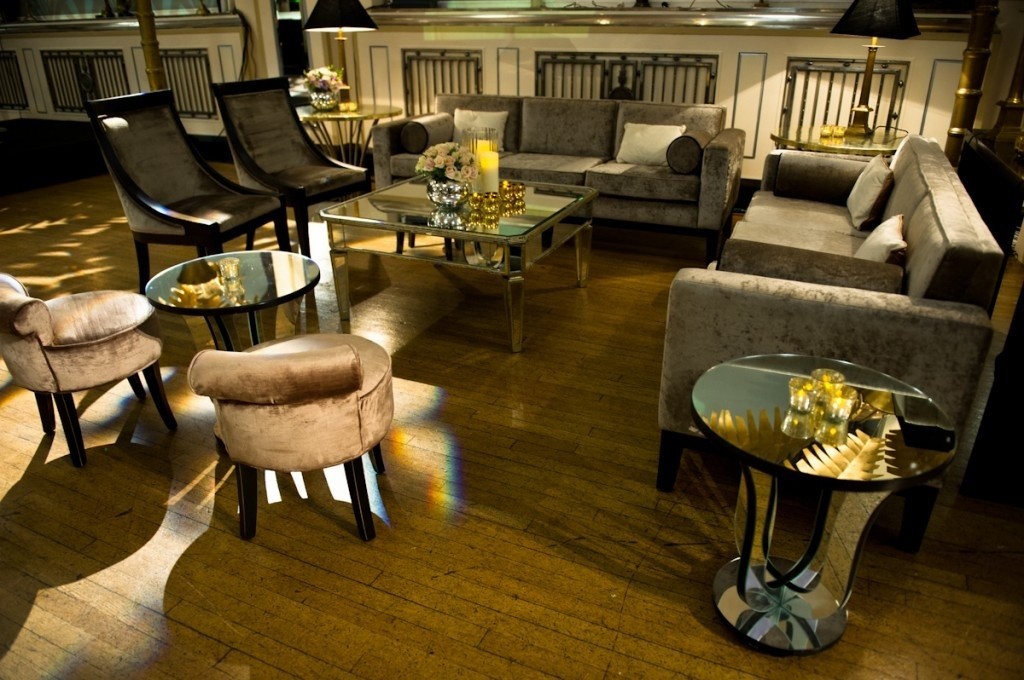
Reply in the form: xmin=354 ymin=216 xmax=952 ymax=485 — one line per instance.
xmin=477 ymin=152 xmax=500 ymax=195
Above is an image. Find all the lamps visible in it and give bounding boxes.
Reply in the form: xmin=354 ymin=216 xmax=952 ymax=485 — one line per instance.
xmin=299 ymin=1 xmax=380 ymax=105
xmin=829 ymin=0 xmax=922 ymax=138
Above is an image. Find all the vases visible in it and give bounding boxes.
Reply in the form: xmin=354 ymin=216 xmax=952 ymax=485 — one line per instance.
xmin=308 ymin=93 xmax=337 ymax=111
xmin=426 ymin=181 xmax=470 ymax=211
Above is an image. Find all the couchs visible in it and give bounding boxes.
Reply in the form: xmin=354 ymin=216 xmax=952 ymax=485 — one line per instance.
xmin=653 ymin=136 xmax=1024 ymax=553
xmin=373 ymin=92 xmax=746 ymax=263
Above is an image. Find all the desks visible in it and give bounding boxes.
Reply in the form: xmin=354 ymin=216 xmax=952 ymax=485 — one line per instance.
xmin=690 ymin=353 xmax=959 ymax=656
xmin=294 ymin=101 xmax=402 ymax=168
xmin=771 ymin=126 xmax=913 ymax=156
xmin=143 ymin=251 xmax=319 ymax=350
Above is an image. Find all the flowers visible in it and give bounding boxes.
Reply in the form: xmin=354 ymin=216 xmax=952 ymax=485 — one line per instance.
xmin=301 ymin=65 xmax=349 ymax=96
xmin=417 ymin=141 xmax=476 ymax=183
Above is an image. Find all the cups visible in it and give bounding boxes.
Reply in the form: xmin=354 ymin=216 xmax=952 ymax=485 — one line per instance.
xmin=468 ymin=198 xmax=527 ymax=231
xmin=338 ymin=101 xmax=358 ymax=112
xmin=788 ymin=368 xmax=859 ymax=424
xmin=469 ymin=181 xmax=525 ymax=214
xmin=218 ymin=257 xmax=240 ymax=280
xmin=224 ymin=277 xmax=245 ymax=297
xmin=779 ymin=402 xmax=848 ymax=448
xmin=820 ymin=124 xmax=846 ymax=138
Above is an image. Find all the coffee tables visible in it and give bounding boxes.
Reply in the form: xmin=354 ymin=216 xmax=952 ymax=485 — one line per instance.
xmin=318 ymin=171 xmax=602 ymax=352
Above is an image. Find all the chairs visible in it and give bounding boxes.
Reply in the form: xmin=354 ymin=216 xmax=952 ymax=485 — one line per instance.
xmin=0 ymin=273 xmax=178 ymax=467
xmin=188 ymin=341 xmax=395 ymax=546
xmin=207 ymin=76 xmax=371 ymax=263
xmin=84 ymin=89 xmax=282 ymax=316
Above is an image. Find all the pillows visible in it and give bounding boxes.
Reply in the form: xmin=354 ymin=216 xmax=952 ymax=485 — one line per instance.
xmin=713 ymin=237 xmax=905 ymax=296
xmin=450 ymin=107 xmax=507 ymax=155
xmin=852 ymin=212 xmax=906 ymax=267
xmin=617 ymin=121 xmax=685 ymax=168
xmin=399 ymin=110 xmax=458 ymax=155
xmin=845 ymin=151 xmax=893 ymax=230
xmin=774 ymin=154 xmax=870 ymax=205
xmin=666 ymin=127 xmax=713 ymax=174
